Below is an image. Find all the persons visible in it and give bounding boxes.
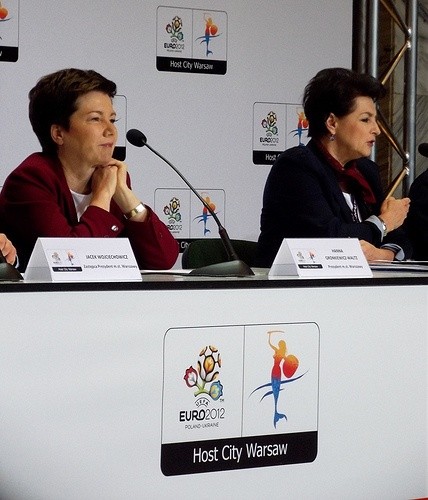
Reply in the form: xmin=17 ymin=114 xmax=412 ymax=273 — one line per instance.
xmin=0 ymin=232 xmax=23 ymax=272
xmin=0 ymin=69 xmax=181 ymax=271
xmin=258 ymin=67 xmax=415 ymax=266
xmin=405 ymin=141 xmax=428 ymax=264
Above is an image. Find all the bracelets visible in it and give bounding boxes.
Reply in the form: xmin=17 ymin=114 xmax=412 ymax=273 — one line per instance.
xmin=377 ymin=217 xmax=387 ymax=236
xmin=124 ymin=202 xmax=145 ymax=221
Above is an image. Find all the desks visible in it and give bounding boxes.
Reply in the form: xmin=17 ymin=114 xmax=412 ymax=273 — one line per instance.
xmin=0 ymin=265 xmax=428 ymax=500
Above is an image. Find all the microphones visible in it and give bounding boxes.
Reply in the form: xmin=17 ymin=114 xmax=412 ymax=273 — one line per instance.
xmin=126 ymin=129 xmax=256 ymax=276
xmin=419 ymin=143 xmax=428 ymax=157
